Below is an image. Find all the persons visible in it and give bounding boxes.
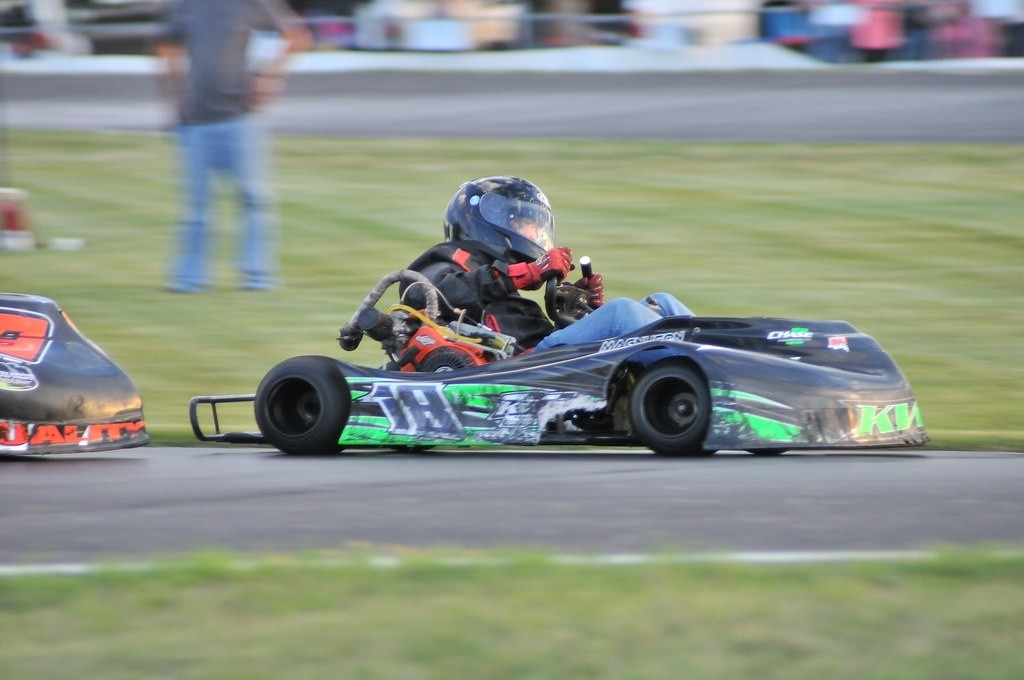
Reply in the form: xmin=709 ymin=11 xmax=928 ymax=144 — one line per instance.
xmin=398 ymin=176 xmax=693 ymax=359
xmin=347 ymin=1 xmax=1023 ymax=65
xmin=0 ymin=1 xmax=288 ymax=59
xmin=151 ymin=0 xmax=313 ymax=292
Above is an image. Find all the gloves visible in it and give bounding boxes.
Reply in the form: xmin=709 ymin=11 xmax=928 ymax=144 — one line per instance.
xmin=573 ymin=275 xmax=603 ymax=307
xmin=508 ymin=247 xmax=573 ymax=289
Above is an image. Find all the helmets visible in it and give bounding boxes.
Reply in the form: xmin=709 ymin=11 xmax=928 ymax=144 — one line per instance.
xmin=444 ymin=176 xmax=554 ymax=264
